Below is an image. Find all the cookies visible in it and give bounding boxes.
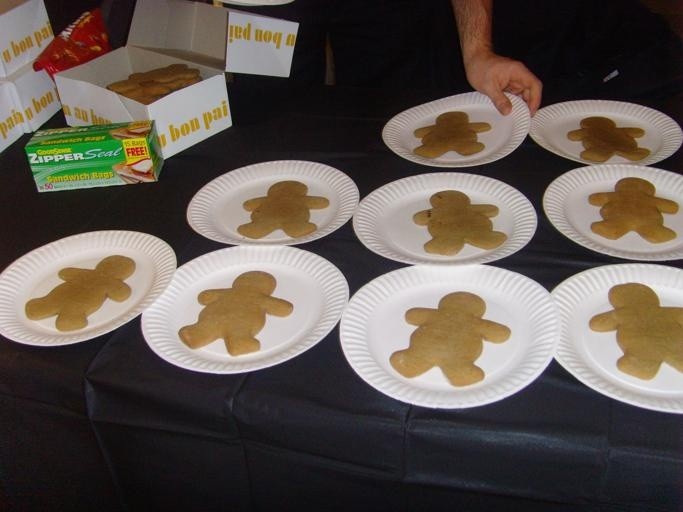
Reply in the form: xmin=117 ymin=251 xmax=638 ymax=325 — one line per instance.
xmin=411 ymin=112 xmax=492 ymax=158
xmin=237 ymin=180 xmax=330 ymax=240
xmin=109 ymin=61 xmax=206 ymax=108
xmin=568 ymin=116 xmax=650 ymax=164
xmin=178 ymin=272 xmax=294 ymax=357
xmin=590 ymin=178 xmax=680 ymax=245
xmin=590 ymin=281 xmax=683 ymax=381
xmin=390 ymin=290 xmax=512 ymax=388
xmin=412 ymin=190 xmax=506 ymax=255
xmin=25 ymin=254 xmax=134 ymax=332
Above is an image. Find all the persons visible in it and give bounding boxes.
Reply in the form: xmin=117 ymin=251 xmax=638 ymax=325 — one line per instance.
xmin=209 ymin=1 xmax=545 ymax=118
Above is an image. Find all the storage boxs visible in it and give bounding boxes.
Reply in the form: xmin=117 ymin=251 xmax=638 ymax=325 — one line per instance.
xmin=24 ymin=119 xmax=166 ymax=193
xmin=121 ymin=2 xmax=300 ymax=80
xmin=2 ymin=1 xmax=63 ymax=164
xmin=50 ymin=44 xmax=235 ymax=162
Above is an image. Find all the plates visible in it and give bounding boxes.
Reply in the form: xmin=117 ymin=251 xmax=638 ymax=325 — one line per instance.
xmin=339 ymin=264 xmax=561 ymax=410
xmin=381 ymin=89 xmax=530 ymax=169
xmin=542 ymin=164 xmax=683 ymax=262
xmin=186 ymin=159 xmax=360 ymax=245
xmin=352 ymin=171 xmax=539 ymax=264
xmin=528 ymin=100 xmax=683 ymax=164
xmin=550 ymin=263 xmax=683 ymax=415
xmin=141 ymin=245 xmax=351 ymax=375
xmin=0 ymin=229 xmax=177 ymax=347
